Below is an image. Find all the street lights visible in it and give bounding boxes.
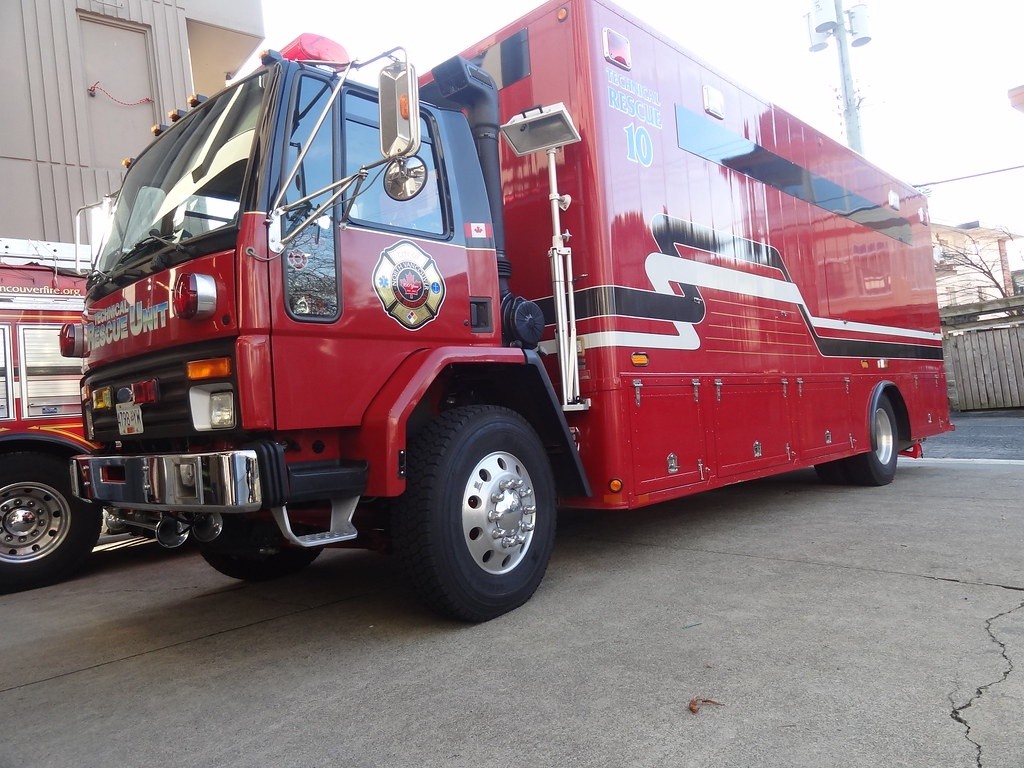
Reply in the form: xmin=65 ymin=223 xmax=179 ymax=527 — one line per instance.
xmin=803 ymin=0 xmax=871 ymax=157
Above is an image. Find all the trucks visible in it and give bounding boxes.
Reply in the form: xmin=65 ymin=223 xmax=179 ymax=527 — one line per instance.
xmin=0 ymin=238 xmax=104 ymax=595
xmin=57 ymin=0 xmax=958 ymax=625
